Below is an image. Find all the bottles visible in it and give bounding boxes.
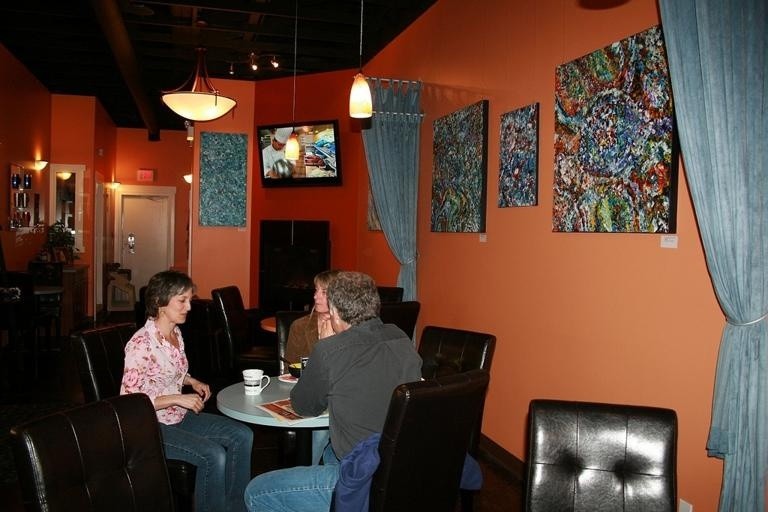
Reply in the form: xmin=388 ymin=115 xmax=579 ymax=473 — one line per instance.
xmin=301 ymin=357 xmax=309 ymax=377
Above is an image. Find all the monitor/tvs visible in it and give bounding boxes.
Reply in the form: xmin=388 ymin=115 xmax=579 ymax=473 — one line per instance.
xmin=256 ymin=119 xmax=342 ymax=188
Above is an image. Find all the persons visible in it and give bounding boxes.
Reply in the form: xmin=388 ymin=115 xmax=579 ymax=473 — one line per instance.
xmin=263 ymin=128 xmax=294 ymax=179
xmin=244 ymin=269 xmax=423 ymax=511
xmin=120 ymin=272 xmax=254 ymax=511
xmin=286 ymin=268 xmax=347 ymax=371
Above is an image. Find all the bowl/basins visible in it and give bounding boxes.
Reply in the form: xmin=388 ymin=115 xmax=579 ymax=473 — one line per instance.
xmin=288 ymin=363 xmax=301 ymax=377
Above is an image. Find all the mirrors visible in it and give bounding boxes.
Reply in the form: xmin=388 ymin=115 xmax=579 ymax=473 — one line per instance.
xmin=49 ymin=164 xmax=86 ymax=254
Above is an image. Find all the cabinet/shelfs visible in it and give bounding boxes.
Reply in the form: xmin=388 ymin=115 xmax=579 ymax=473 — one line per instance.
xmin=60 ymin=264 xmax=88 ymax=335
xmin=9 ymin=162 xmax=33 ymax=231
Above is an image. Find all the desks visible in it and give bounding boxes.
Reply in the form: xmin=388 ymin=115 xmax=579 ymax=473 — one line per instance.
xmin=32 ymin=288 xmax=65 ymax=336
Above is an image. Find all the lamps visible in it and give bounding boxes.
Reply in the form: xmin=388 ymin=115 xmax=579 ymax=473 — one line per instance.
xmin=349 ymin=0 xmax=373 ymax=118
xmin=161 ymin=18 xmax=236 ymax=123
xmin=285 ymin=1 xmax=300 ymax=160
xmin=228 ymin=50 xmax=279 ymax=75
xmin=57 ymin=172 xmax=72 ymax=180
xmin=35 ymin=160 xmax=49 ymax=171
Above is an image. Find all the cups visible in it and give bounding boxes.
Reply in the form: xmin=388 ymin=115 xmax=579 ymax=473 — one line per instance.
xmin=22 ymin=192 xmax=29 ymax=207
xmin=13 ymin=174 xmax=21 ymax=189
xmin=14 ymin=192 xmax=19 ymax=206
xmin=242 ymin=369 xmax=270 ymax=396
xmin=23 ymin=212 xmax=30 ymax=227
xmin=23 ymin=174 xmax=32 ymax=189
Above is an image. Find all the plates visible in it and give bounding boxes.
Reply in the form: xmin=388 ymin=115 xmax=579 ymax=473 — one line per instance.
xmin=278 ymin=373 xmax=299 ymax=383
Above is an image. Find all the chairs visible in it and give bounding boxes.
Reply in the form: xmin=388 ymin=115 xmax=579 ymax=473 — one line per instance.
xmin=524 ymin=399 xmax=678 ymax=511
xmin=7 ymin=394 xmax=174 ymax=511
xmin=375 ymin=287 xmax=498 ymax=380
xmin=29 ymin=260 xmax=63 ymax=341
xmin=62 ymin=323 xmax=197 ymax=496
xmin=369 ymin=368 xmax=490 ymax=510
xmin=187 ymin=282 xmax=314 ymax=378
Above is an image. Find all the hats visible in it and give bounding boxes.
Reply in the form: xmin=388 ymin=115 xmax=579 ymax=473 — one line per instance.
xmin=273 ymin=127 xmax=294 ymax=144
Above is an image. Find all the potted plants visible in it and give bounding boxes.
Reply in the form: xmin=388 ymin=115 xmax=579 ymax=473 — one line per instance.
xmin=49 ymin=219 xmax=75 ymax=263
